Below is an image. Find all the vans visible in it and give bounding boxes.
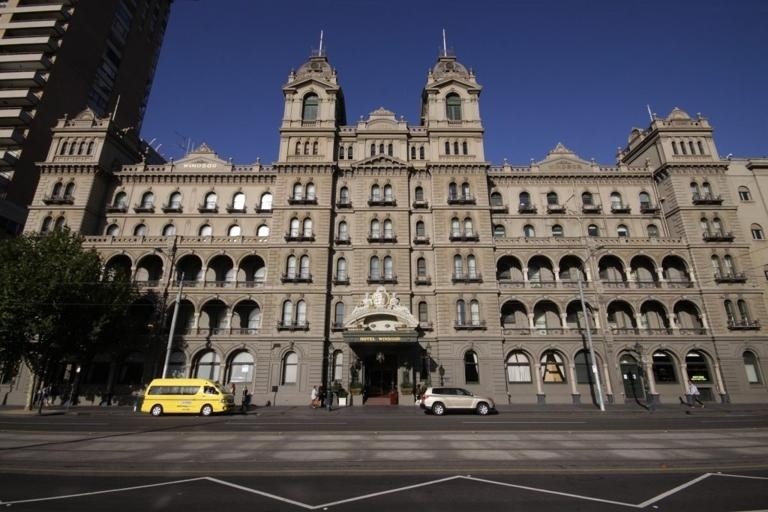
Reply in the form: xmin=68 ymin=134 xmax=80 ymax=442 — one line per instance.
xmin=141 ymin=378 xmax=235 ymax=416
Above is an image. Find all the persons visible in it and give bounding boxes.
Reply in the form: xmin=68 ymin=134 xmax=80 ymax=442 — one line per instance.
xmin=230 ymin=383 xmax=236 ymax=396
xmin=240 ymin=384 xmax=249 ymax=411
xmin=688 ymin=380 xmax=705 ymax=409
xmin=415 ymin=383 xmax=428 ymax=400
xmin=38 ymin=387 xmax=50 ymax=407
xmin=311 ymin=382 xmax=325 ymax=408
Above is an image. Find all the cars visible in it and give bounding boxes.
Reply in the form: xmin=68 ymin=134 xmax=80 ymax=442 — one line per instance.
xmin=420 ymin=386 xmax=495 ymax=416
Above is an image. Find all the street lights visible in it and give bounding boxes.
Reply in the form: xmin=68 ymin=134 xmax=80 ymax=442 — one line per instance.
xmin=576 ymin=245 xmax=606 ymax=412
xmin=155 ymin=248 xmax=185 ymax=378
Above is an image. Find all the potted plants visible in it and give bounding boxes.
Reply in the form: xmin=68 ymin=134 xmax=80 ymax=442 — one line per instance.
xmin=349 ymin=382 xmax=363 ymax=394
xmin=399 ymin=382 xmax=414 ymax=394
